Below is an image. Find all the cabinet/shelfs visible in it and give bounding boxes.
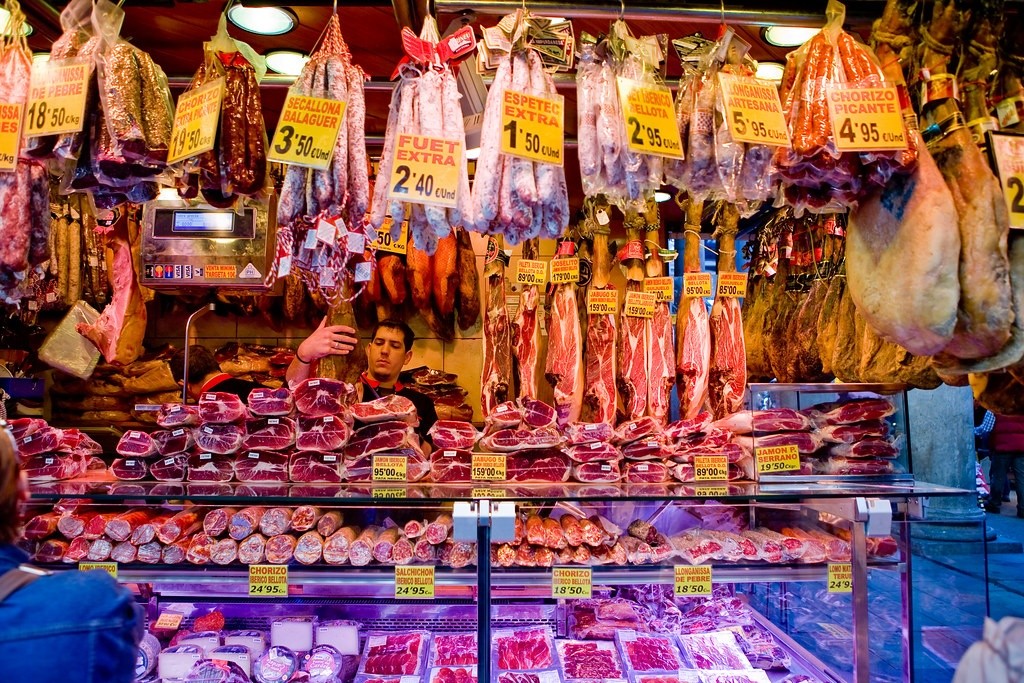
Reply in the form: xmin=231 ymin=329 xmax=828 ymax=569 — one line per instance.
xmin=22 ymin=481 xmax=991 ymax=683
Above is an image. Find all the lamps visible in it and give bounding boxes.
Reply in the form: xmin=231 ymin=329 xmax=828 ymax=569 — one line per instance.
xmin=265 ymin=50 xmax=313 ymax=75
xmin=760 ymin=26 xmax=822 ymax=48
xmin=755 ymin=62 xmax=785 ymax=82
xmin=226 ymin=3 xmax=299 ymax=37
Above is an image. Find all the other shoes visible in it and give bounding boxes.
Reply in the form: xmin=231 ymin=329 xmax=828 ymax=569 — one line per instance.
xmin=1001 ymin=495 xmax=1010 ymax=502
xmin=1016 ymin=506 xmax=1024 ymax=518
xmin=984 ymin=500 xmax=1001 ymax=514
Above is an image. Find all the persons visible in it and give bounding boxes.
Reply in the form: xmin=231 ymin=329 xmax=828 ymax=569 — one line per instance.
xmin=0 ymin=429 xmax=143 ymax=683
xmin=286 ymin=316 xmax=437 ymax=460
xmin=173 ymin=345 xmax=276 ymax=409
xmin=974 ymin=385 xmax=1024 ymax=518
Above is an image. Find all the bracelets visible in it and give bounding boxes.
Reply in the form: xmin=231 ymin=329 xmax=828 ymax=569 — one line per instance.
xmin=295 ymin=351 xmax=312 ymax=365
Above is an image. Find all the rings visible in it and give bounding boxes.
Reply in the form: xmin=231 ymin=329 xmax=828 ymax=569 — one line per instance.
xmin=335 ymin=342 xmax=338 ymax=349
